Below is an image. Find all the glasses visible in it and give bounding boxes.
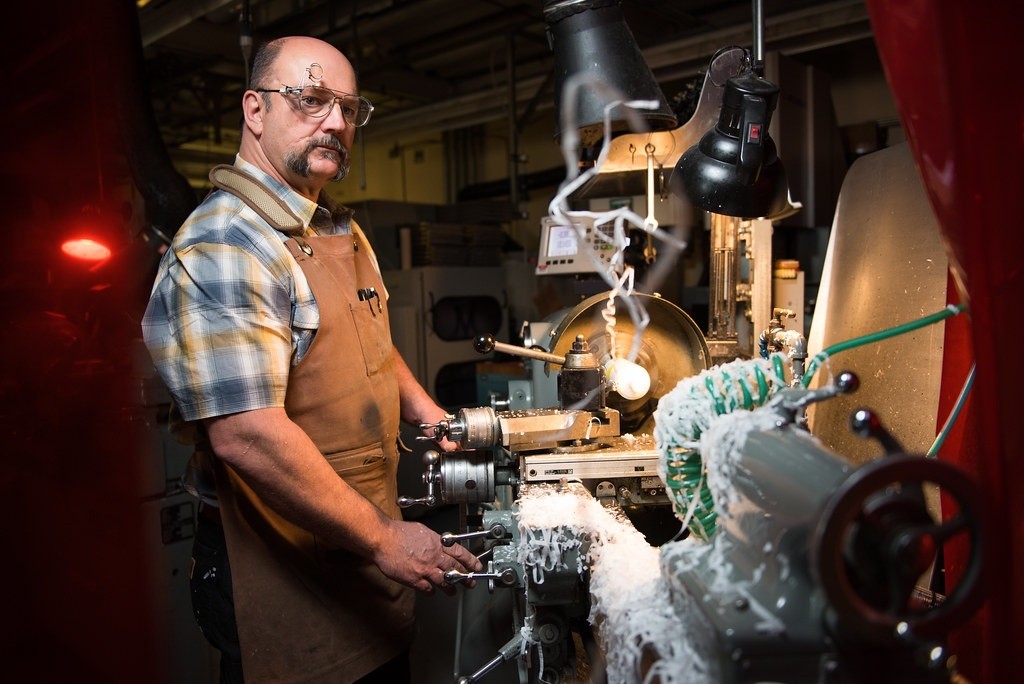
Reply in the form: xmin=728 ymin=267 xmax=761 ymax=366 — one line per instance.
xmin=255 ymin=62 xmax=375 ymax=129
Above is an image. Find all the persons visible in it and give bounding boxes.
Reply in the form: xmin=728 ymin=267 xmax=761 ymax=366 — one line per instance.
xmin=141 ymin=36 xmax=482 ymax=684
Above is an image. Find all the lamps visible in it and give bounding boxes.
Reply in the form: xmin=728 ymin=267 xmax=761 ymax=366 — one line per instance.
xmin=666 ymin=0 xmax=788 ymax=218
